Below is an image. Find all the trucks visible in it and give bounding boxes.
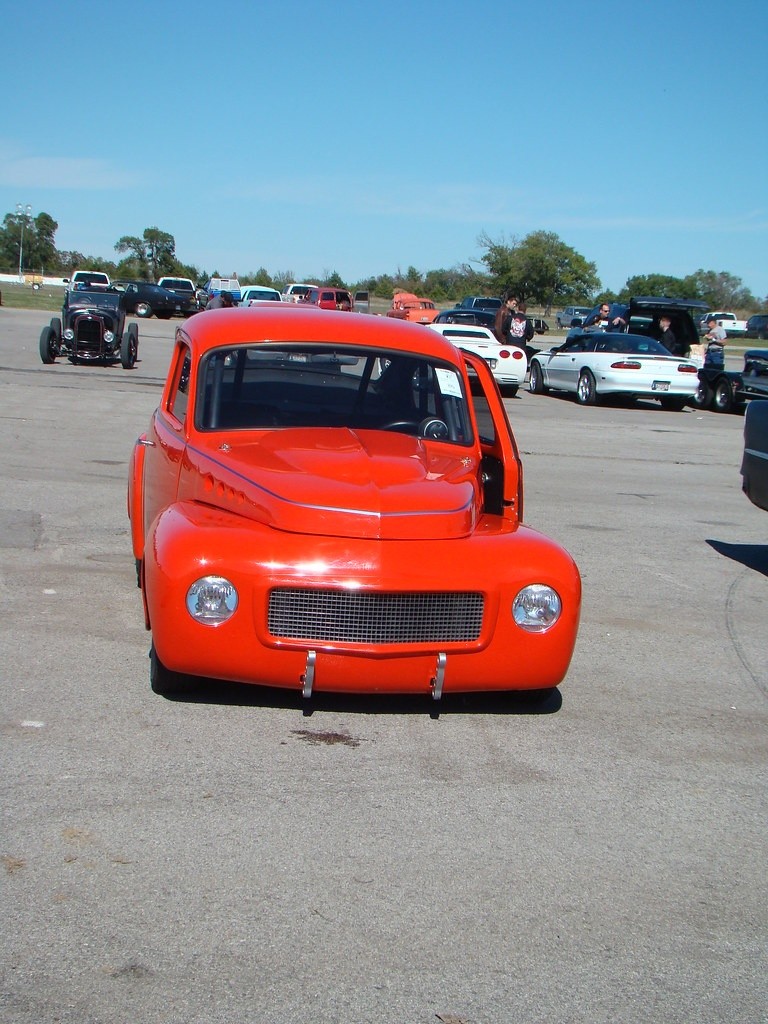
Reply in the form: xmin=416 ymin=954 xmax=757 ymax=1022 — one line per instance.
xmin=689 ymin=348 xmax=768 ymax=410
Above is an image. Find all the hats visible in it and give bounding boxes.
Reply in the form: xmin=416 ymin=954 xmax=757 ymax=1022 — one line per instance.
xmin=703 ymin=314 xmax=717 ymax=324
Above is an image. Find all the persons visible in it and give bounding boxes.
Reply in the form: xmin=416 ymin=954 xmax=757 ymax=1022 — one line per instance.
xmin=567 ymin=304 xmax=625 ymax=337
xmin=503 ymin=303 xmax=534 ymax=346
xmin=204 ymin=290 xmax=235 ymax=311
xmin=702 ymin=316 xmax=728 ymax=364
xmin=494 ymin=296 xmax=518 ymax=343
xmin=658 ymin=317 xmax=676 ymax=353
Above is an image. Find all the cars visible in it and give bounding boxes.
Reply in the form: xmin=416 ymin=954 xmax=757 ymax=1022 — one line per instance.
xmin=741 ymin=399 xmax=768 ymax=512
xmin=126 ymin=306 xmax=581 ymax=701
xmin=40 ymin=282 xmax=189 ymax=369
xmin=385 ymin=293 xmax=440 ymax=324
xmin=434 ymin=310 xmax=503 ymax=342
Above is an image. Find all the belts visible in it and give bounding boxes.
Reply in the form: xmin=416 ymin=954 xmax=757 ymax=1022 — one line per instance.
xmin=711 ymin=349 xmax=723 ymax=352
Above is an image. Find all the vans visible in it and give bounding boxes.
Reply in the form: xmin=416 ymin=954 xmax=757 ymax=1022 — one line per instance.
xmin=295 ymin=287 xmax=370 ymax=313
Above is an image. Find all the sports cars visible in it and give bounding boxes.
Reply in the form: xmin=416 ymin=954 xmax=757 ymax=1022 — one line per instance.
xmin=528 ymin=330 xmax=699 ymax=412
xmin=378 ymin=323 xmax=528 ymax=396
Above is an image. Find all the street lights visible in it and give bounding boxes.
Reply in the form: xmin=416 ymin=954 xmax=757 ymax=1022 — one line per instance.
xmin=15 ymin=203 xmax=32 ymax=283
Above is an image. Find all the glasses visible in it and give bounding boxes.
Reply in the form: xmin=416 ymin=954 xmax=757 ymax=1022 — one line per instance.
xmin=601 ymin=309 xmax=610 ymax=313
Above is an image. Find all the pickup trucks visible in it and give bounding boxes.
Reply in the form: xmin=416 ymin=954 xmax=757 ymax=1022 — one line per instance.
xmin=158 ymin=277 xmax=199 ymax=309
xmin=280 ymin=284 xmax=318 ymax=304
xmin=697 ymin=310 xmax=748 ymax=337
xmin=454 ymin=297 xmax=503 ymax=314
xmin=555 ymin=307 xmax=593 ymax=329
xmin=239 ymin=285 xmax=282 ymax=307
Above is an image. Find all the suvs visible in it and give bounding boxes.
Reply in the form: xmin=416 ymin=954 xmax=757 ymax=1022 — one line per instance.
xmin=745 ymin=315 xmax=768 ymax=339
xmin=63 ymin=271 xmax=110 ymax=301
xmin=195 ymin=278 xmax=241 ymax=306
xmin=582 ymin=295 xmax=710 ymax=357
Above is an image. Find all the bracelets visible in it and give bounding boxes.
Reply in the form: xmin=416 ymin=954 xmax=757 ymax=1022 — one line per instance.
xmin=712 ymin=337 xmax=717 ymax=342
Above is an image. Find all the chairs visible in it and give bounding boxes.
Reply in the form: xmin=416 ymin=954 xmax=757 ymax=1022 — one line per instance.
xmin=128 ymin=285 xmax=133 ymax=291
